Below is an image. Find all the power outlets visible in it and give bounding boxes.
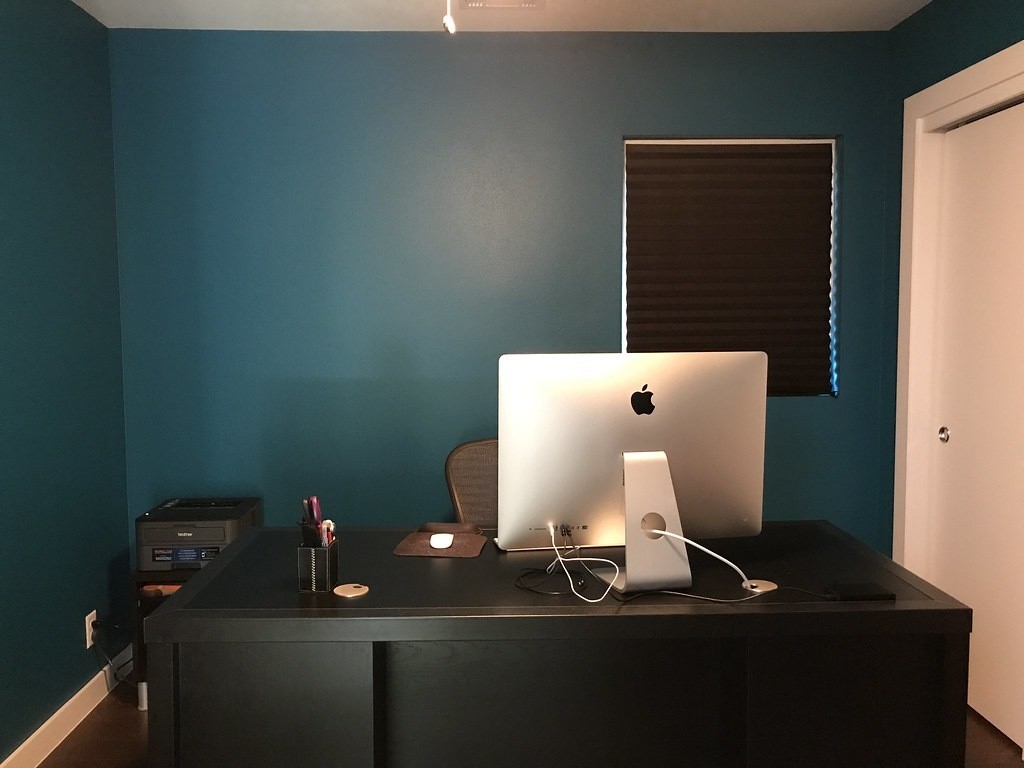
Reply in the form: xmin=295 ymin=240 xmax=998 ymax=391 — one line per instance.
xmin=85 ymin=610 xmax=96 ymax=649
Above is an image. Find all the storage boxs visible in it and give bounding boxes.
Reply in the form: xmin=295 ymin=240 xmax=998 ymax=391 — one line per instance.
xmin=135 ymin=497 xmax=262 ymax=570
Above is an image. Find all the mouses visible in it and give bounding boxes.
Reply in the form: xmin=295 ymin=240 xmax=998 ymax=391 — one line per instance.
xmin=430 ymin=533 xmax=454 ymax=548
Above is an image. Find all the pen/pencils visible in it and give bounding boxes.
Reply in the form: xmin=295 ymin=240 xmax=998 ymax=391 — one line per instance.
xmin=301 ymin=494 xmax=338 ymax=551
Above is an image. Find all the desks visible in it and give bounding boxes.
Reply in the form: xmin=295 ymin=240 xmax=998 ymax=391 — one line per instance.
xmin=143 ymin=521 xmax=974 ymax=768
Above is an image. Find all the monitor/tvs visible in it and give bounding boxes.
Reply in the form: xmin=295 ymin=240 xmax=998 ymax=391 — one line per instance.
xmin=498 ymin=352 xmax=768 ymax=593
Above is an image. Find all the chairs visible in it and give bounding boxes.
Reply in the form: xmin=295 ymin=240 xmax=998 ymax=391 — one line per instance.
xmin=444 ymin=439 xmax=498 ymax=525
